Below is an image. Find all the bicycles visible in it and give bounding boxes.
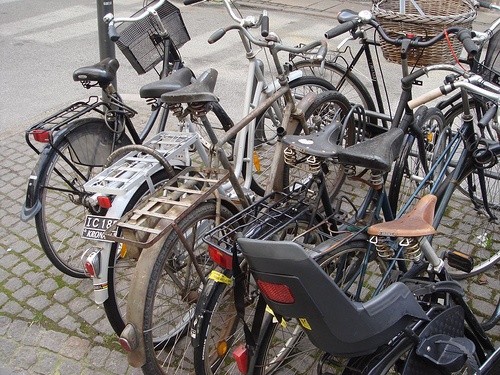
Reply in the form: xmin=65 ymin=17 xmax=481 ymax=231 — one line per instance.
xmin=19 ymin=0 xmax=500 ymax=375
